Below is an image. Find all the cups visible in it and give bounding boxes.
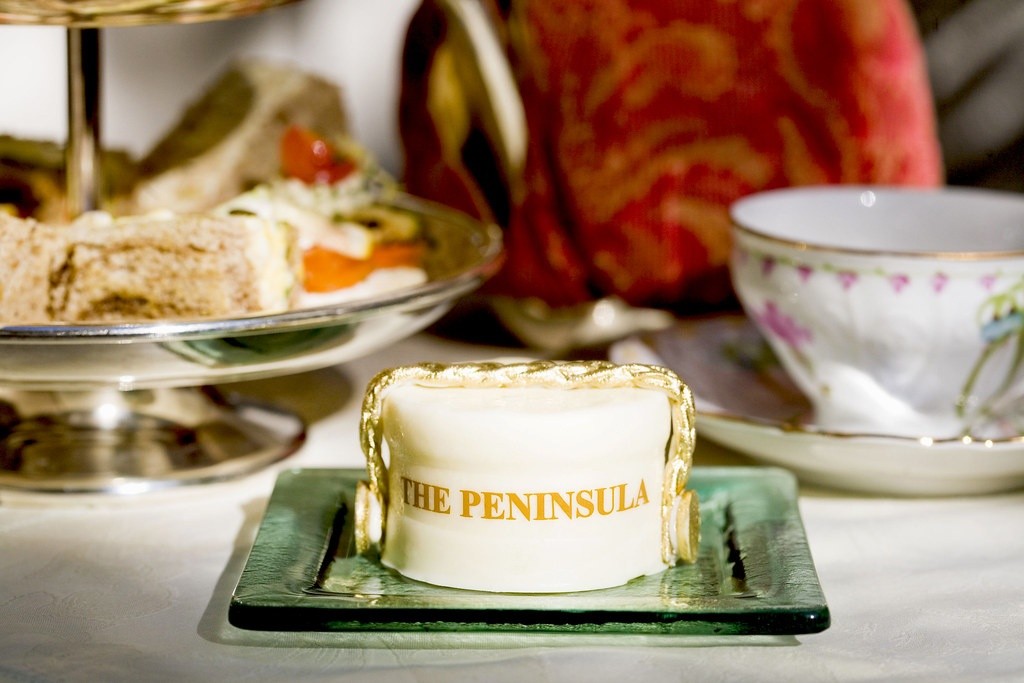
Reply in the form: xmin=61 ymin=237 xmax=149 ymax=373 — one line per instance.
xmin=731 ymin=185 xmax=1024 ymax=440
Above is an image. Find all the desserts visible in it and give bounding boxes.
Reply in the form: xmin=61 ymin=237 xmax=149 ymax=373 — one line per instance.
xmin=0 ymin=204 xmax=297 ymax=328
xmin=342 ymin=357 xmax=700 ymax=592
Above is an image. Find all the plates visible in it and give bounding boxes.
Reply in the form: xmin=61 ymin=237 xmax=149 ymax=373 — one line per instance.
xmin=611 ymin=316 xmax=1024 ymax=497
xmin=227 ymin=467 xmax=831 ymax=636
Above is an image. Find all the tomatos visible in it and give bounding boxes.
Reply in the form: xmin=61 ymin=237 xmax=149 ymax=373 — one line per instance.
xmin=279 ymin=123 xmax=351 ymax=182
